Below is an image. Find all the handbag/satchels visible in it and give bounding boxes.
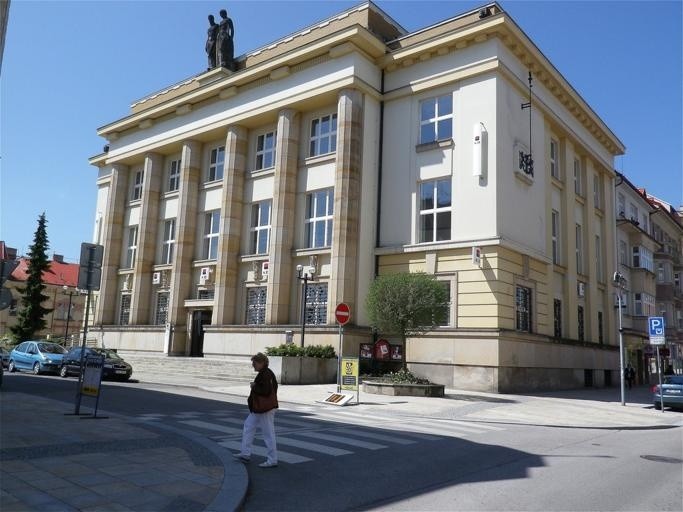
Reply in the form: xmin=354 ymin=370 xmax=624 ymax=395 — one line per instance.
xmin=251 ymin=370 xmax=278 ymax=413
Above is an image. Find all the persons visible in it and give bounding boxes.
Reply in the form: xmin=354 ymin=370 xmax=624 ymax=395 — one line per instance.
xmin=217 ymin=9 xmax=234 ymax=66
xmin=204 ymin=15 xmax=220 ymax=69
xmin=232 ymin=352 xmax=279 ymax=469
xmin=625 ymin=363 xmax=634 ymax=390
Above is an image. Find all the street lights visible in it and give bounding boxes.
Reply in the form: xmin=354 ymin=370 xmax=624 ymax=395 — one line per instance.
xmin=612 ymin=268 xmax=627 ymax=407
xmin=60 ymin=284 xmax=80 ymax=345
xmin=295 ymin=264 xmax=316 ymax=349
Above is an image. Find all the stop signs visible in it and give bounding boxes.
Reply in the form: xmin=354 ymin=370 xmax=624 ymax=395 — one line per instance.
xmin=335 ymin=303 xmax=350 ymax=325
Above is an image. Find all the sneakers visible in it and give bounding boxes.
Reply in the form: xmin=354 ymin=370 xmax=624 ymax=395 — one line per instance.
xmin=231 ymin=451 xmax=252 ymax=462
xmin=257 ymin=460 xmax=280 ymax=469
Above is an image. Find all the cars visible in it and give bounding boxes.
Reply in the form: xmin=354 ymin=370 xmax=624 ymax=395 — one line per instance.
xmin=59 ymin=346 xmax=132 ymax=382
xmin=7 ymin=341 xmax=70 ymax=374
xmin=0 ymin=346 xmax=12 ymax=368
xmin=650 ymin=374 xmax=683 ymax=413
